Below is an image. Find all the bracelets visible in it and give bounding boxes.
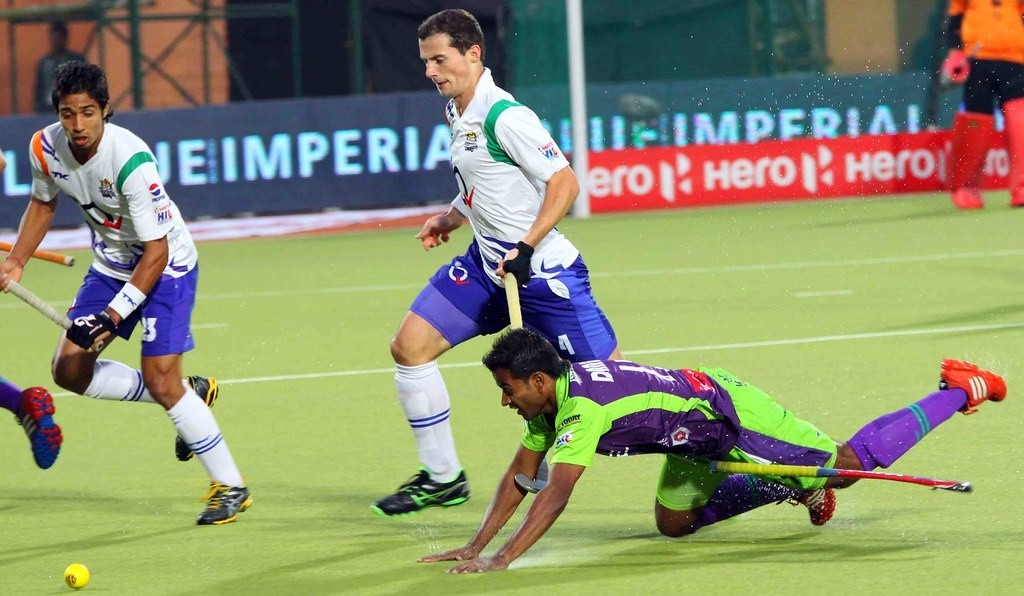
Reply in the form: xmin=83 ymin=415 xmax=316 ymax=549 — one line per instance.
xmin=6 ymin=256 xmax=24 ymax=269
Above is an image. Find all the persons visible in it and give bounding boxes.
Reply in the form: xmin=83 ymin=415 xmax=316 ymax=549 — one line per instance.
xmin=940 ymin=0 xmax=1024 ymax=211
xmin=0 ymin=61 xmax=252 ymax=524
xmin=371 ymin=11 xmax=621 ymax=519
xmin=417 ymin=330 xmax=1007 ymax=575
xmin=33 ymin=21 xmax=88 ymax=115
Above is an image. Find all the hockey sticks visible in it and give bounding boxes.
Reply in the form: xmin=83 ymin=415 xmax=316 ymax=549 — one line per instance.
xmin=708 ymin=461 xmax=973 ymax=494
xmin=503 ymin=272 xmax=549 ymax=494
xmin=5 ymin=280 xmax=74 ymax=331
xmin=0 ymin=242 xmax=75 ymax=267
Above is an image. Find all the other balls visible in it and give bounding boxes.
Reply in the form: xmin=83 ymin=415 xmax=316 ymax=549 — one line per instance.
xmin=64 ymin=563 xmax=91 ymax=589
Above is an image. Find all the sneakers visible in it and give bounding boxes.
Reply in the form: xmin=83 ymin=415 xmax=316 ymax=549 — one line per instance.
xmin=15 ymin=387 xmax=63 ymax=469
xmin=786 ymin=487 xmax=836 ymax=525
xmin=197 ymin=475 xmax=252 ymax=525
xmin=939 ymin=358 xmax=1008 ymax=415
xmin=370 ymin=466 xmax=471 ymax=519
xmin=175 ymin=375 xmax=219 ymax=461
